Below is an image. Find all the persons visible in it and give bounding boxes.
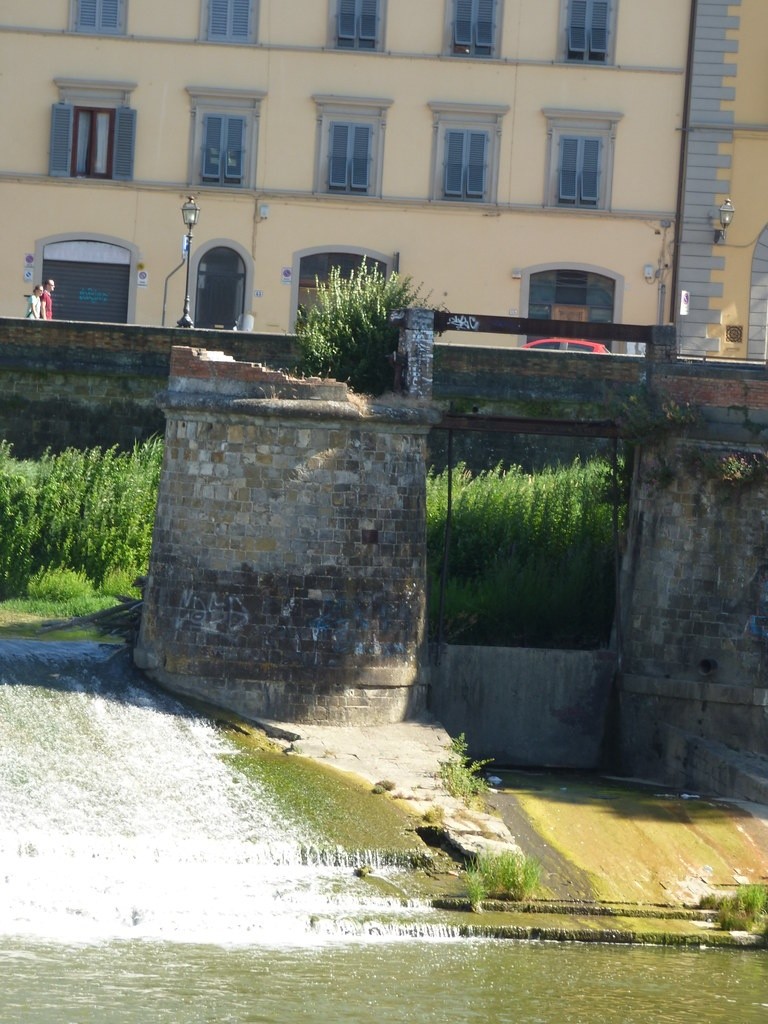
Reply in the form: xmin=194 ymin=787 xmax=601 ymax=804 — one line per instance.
xmin=26 ymin=285 xmax=44 ymax=319
xmin=40 ymin=279 xmax=55 ymax=319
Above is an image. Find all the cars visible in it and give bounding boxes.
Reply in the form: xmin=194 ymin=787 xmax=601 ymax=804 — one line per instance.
xmin=522 ymin=339 xmax=612 ymax=357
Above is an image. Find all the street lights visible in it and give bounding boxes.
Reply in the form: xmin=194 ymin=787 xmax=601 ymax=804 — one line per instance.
xmin=173 ymin=193 xmax=204 ymax=325
